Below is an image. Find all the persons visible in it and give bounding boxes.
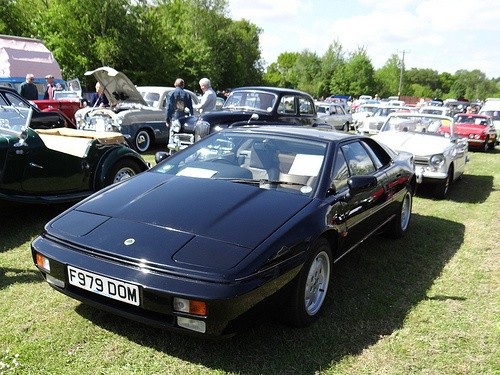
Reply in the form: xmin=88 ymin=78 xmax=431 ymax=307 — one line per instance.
xmin=43 ymin=75 xmax=63 ymax=100
xmin=165 ymin=78 xmax=231 ymax=155
xmin=90 ymin=81 xmax=109 ymax=108
xmin=17 ymin=73 xmax=38 ymax=101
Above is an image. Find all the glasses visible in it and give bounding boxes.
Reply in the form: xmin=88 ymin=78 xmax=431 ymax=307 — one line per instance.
xmin=31 ymin=79 xmax=34 ymax=80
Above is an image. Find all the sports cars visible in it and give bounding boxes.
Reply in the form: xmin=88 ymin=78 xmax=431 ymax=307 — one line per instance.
xmin=370 ymin=113 xmax=471 ymax=200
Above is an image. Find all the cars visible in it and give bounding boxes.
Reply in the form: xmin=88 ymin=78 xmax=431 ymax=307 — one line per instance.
xmin=0 ymin=102 xmax=152 ymax=206
xmin=1 ymin=76 xmax=500 ymax=153
xmin=28 ymin=123 xmax=417 ymax=339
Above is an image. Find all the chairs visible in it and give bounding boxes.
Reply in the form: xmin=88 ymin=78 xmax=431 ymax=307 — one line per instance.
xmin=236 ymin=142 xmax=321 ymax=196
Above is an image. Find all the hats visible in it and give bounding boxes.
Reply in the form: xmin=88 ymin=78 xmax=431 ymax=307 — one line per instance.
xmin=45 ymin=74 xmax=54 ymax=78
